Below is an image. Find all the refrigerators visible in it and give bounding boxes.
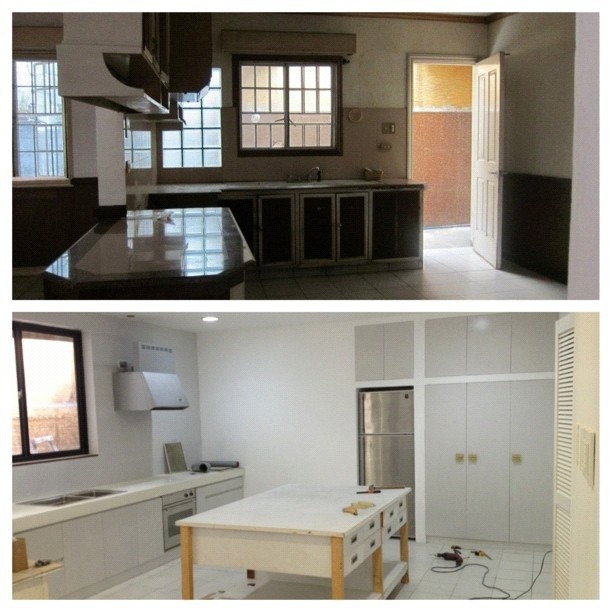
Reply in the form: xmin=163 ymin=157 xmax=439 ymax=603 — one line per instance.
xmin=357 ymin=389 xmax=415 ymax=540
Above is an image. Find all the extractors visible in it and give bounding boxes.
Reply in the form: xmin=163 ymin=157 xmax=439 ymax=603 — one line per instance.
xmin=114 ymin=371 xmax=191 ymax=412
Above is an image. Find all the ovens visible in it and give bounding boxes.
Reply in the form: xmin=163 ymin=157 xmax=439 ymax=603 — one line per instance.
xmin=160 ymin=488 xmax=197 ymax=552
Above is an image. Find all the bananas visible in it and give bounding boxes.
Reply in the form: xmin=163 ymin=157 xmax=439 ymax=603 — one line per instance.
xmin=342 ymin=506 xmax=358 ymax=516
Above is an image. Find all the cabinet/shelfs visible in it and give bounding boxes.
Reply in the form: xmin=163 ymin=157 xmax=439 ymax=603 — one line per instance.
xmin=53 ymin=44 xmax=171 ymax=116
xmin=298 ymin=191 xmax=370 ymax=268
xmin=354 ymin=321 xmax=413 ymax=382
xmin=424 ymin=313 xmax=512 ymax=377
xmin=221 ymin=193 xmax=297 ymax=273
xmin=425 ymin=380 xmax=510 ymax=543
xmin=370 ymin=187 xmax=422 ymax=263
xmin=510 ymin=312 xmax=554 ymax=373
xmin=509 ymin=378 xmax=553 ymax=545
xmin=197 ymin=477 xmax=245 ymax=514
xmin=13 ymin=512 xmax=103 ymax=599
xmin=62 ymin=12 xmax=170 ymax=86
xmin=104 ymin=498 xmax=165 ymax=582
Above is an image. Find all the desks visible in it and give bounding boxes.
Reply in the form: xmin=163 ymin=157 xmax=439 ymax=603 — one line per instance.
xmin=42 ymin=203 xmax=255 ymax=299
xmin=173 ymin=484 xmax=411 ymax=600
xmin=13 ymin=559 xmax=67 ymax=599
